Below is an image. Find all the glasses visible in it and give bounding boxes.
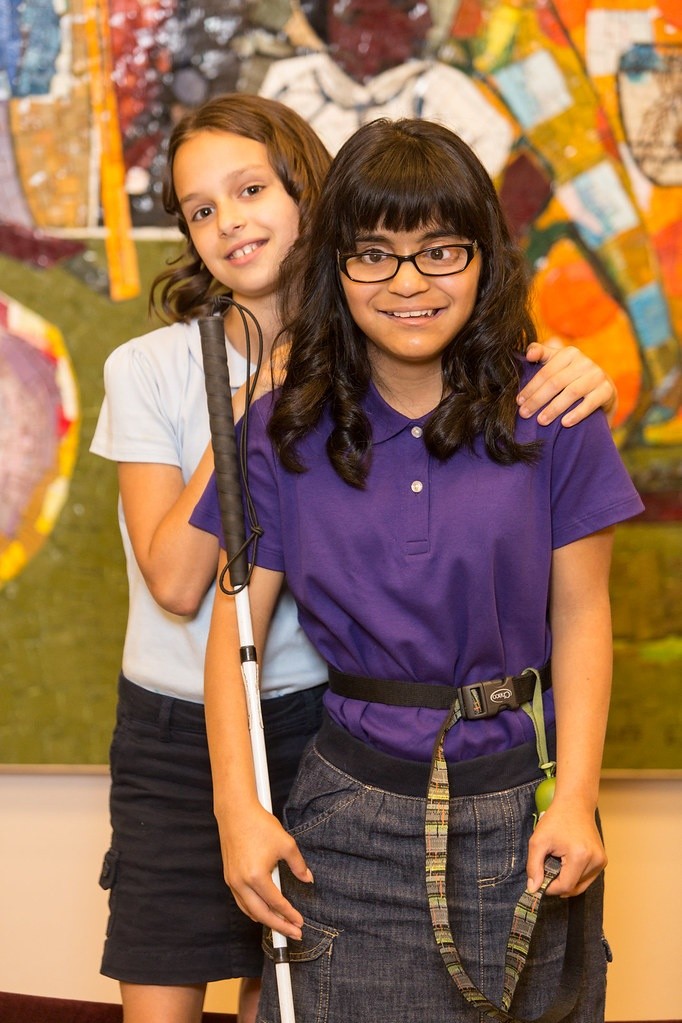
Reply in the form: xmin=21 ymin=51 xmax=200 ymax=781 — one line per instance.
xmin=335 ymin=237 xmax=480 ymax=282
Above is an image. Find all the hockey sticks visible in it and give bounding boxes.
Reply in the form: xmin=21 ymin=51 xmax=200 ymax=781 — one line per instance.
xmin=195 ymin=314 xmax=297 ymax=1023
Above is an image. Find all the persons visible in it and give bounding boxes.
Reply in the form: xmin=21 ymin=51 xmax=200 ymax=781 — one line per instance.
xmin=89 ymin=94 xmax=617 ymax=1022
xmin=189 ymin=118 xmax=645 ymax=1022
